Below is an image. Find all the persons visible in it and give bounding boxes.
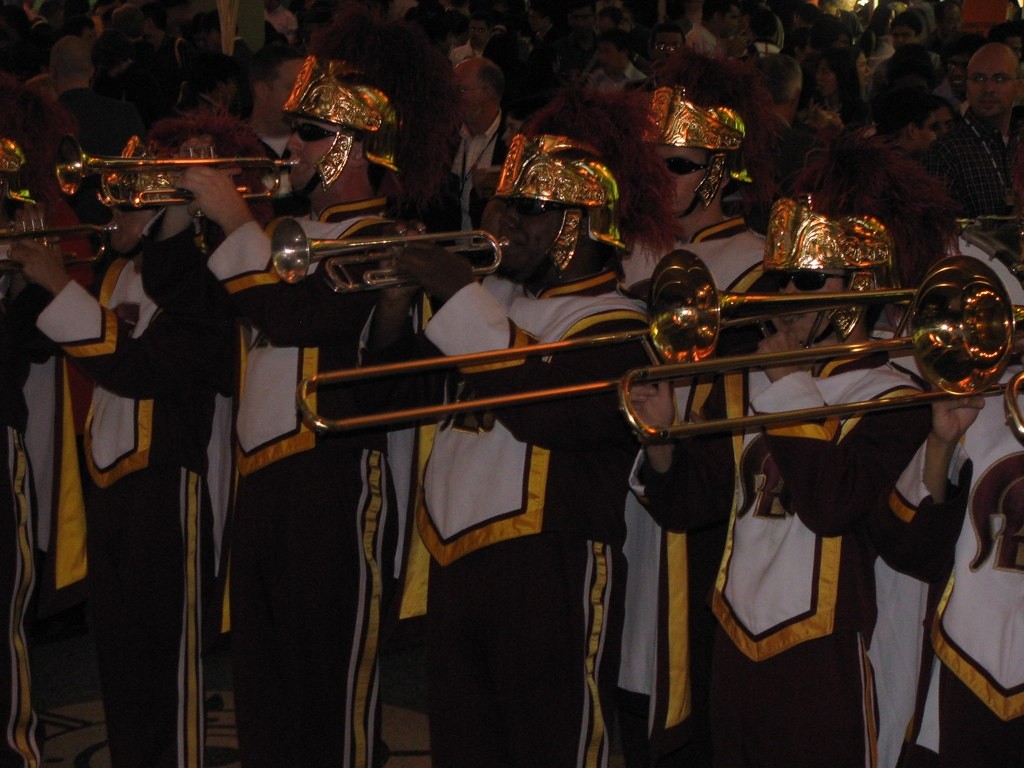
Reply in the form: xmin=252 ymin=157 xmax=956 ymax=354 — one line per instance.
xmin=0 ymin=1 xmax=1024 ymax=768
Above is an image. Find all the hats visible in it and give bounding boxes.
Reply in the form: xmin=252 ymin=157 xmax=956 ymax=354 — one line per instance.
xmin=495 ymin=133 xmax=626 ymax=274
xmin=282 ymin=54 xmax=400 ymax=192
xmin=640 ymin=85 xmax=745 ymax=208
xmin=764 ymin=192 xmax=893 ymax=344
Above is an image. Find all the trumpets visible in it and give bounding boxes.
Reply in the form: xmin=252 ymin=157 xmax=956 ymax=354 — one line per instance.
xmin=0 ymin=220 xmax=119 ymax=268
xmin=262 ymin=218 xmax=512 ymax=300
xmin=53 ymin=134 xmax=302 ymax=206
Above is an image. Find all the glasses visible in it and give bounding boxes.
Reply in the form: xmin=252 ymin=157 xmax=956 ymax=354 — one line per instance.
xmin=665 ymin=156 xmax=708 ymax=175
xmin=653 ymin=40 xmax=681 ymax=54
xmin=290 ymin=122 xmax=337 ymax=142
xmin=469 ymin=27 xmax=487 ymax=33
xmin=775 ymin=270 xmax=848 ymax=291
xmin=453 ymin=87 xmax=483 ymax=96
xmin=572 ymin=14 xmax=591 ymax=20
xmin=500 ymin=197 xmax=567 ymax=217
xmin=967 ymin=74 xmax=1019 ymax=84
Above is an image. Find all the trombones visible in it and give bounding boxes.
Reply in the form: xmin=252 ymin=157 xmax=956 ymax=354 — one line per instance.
xmin=297 ymin=250 xmax=1024 ymax=453
